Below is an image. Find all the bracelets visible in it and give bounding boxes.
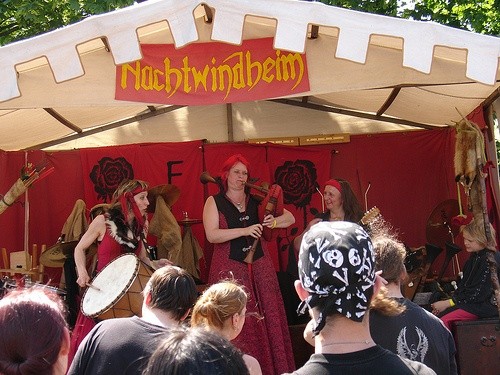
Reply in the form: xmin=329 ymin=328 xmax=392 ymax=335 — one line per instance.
xmin=271 ymin=219 xmax=276 ymax=229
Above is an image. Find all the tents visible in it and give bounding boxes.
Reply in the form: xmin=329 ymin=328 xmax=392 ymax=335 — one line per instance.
xmin=1 ymin=0 xmax=499 ymax=279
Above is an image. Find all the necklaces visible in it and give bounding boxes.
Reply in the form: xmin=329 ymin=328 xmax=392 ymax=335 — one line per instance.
xmin=228 ymin=194 xmax=245 ymax=209
xmin=320 ymin=340 xmax=374 ymax=346
xmin=330 ymin=215 xmax=341 ymax=220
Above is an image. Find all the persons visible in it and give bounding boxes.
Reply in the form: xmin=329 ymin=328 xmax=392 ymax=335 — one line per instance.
xmin=294 ymin=174 xmax=364 ymax=250
xmin=74 ymin=179 xmax=175 ymax=375
xmin=289 ymin=219 xmax=442 ymax=375
xmin=145 ymin=327 xmax=252 ymax=375
xmin=438 ymin=209 xmax=500 ymax=338
xmin=190 ymin=277 xmax=265 ymax=375
xmin=366 ymin=229 xmax=460 ymax=375
xmin=0 ymin=284 xmax=72 ymax=375
xmin=65 ymin=266 xmax=198 ymax=375
xmin=197 ymin=153 xmax=297 ymax=375
